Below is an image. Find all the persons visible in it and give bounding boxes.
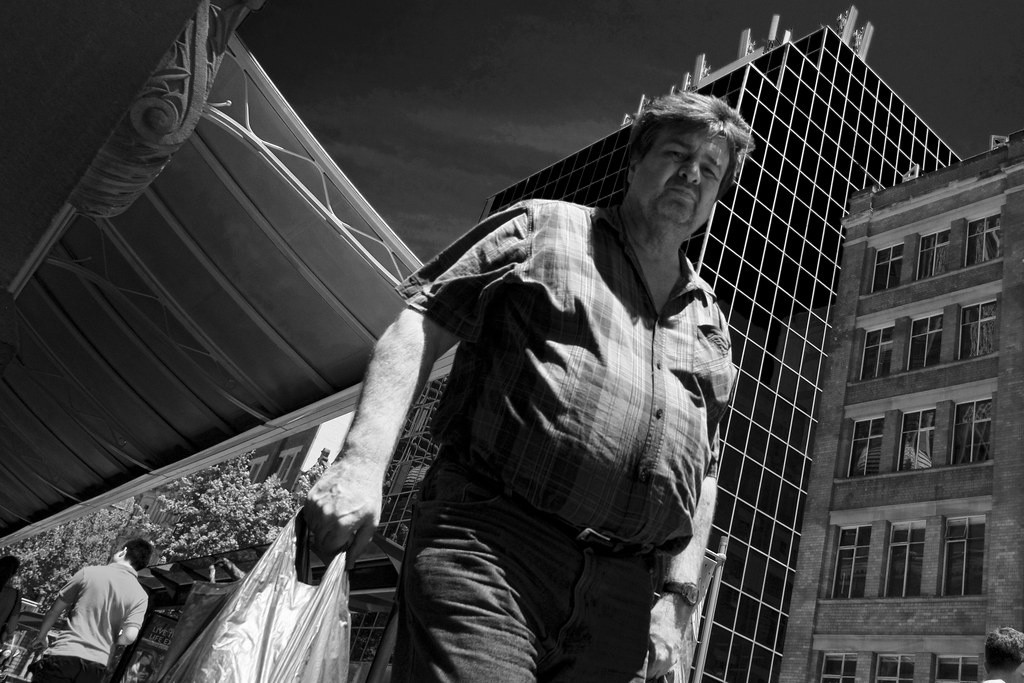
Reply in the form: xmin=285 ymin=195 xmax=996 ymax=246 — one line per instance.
xmin=982 ymin=625 xmax=1024 ymax=683
xmin=0 ymin=555 xmax=22 ymax=644
xmin=30 ymin=538 xmax=153 ymax=683
xmin=303 ymin=91 xmax=756 ymax=683
xmin=0 ymin=648 xmax=11 ymax=667
xmin=136 ymin=651 xmax=155 ymax=683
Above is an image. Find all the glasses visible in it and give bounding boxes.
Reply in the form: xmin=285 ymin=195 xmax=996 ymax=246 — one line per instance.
xmin=136 ymin=663 xmax=152 ymax=673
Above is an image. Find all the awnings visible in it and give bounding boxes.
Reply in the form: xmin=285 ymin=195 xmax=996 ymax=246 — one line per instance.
xmin=1 ymin=34 xmax=464 ymax=555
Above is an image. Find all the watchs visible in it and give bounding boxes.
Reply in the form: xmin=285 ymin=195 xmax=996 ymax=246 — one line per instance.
xmin=662 ymin=577 xmax=699 ymax=607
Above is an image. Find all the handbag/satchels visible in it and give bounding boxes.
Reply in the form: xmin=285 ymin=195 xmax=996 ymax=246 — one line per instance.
xmin=0 ymin=624 xmax=9 ymax=644
xmin=151 ymin=505 xmax=351 ymax=683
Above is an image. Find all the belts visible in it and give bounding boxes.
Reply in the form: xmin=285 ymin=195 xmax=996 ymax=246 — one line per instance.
xmin=464 ymin=469 xmax=651 ymax=560
